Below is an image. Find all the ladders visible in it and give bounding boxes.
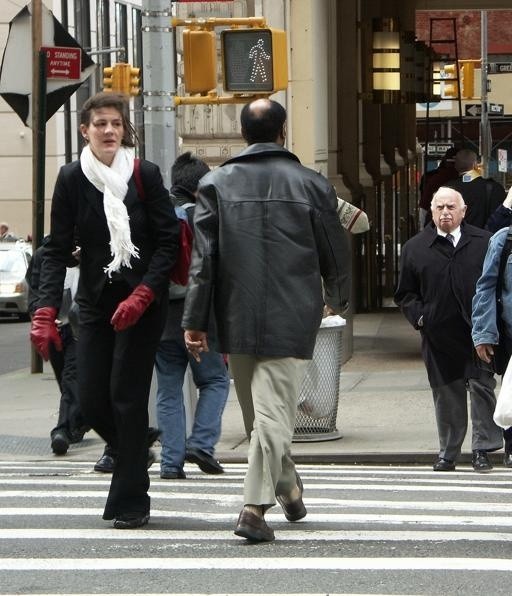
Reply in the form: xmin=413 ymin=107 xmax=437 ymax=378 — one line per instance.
xmin=423 ymin=15 xmax=465 ymax=178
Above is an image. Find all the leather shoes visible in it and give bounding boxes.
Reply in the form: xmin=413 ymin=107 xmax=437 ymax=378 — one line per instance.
xmin=114 ymin=513 xmax=150 ymax=528
xmin=472 ymin=450 xmax=492 ymax=470
xmin=94 ymin=446 xmax=115 ymax=472
xmin=504 ymin=452 xmax=512 ymax=467
xmin=433 ymin=458 xmax=456 ymax=472
xmin=235 ymin=510 xmax=276 ymax=542
xmin=185 ymin=447 xmax=224 ymax=474
xmin=274 ymin=473 xmax=308 ymax=522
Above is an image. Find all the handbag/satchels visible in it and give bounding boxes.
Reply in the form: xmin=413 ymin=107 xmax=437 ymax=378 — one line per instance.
xmin=473 ymin=305 xmax=511 ymax=375
xmin=166 ymin=203 xmax=194 ymax=299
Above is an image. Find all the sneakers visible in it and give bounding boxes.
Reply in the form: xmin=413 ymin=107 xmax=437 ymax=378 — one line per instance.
xmin=51 ymin=432 xmax=69 ymax=455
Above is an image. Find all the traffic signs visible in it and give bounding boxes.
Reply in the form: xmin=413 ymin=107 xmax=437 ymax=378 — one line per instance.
xmin=463 ymin=101 xmax=507 ymax=118
xmin=421 ymin=143 xmax=454 ymax=155
xmin=487 ymin=61 xmax=512 ymax=73
xmin=40 ymin=46 xmax=81 ymax=80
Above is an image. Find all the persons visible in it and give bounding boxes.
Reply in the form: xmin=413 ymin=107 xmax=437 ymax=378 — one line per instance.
xmin=393 ymin=186 xmax=505 ymax=472
xmin=24 ymin=227 xmax=93 ymax=452
xmin=0 ymin=224 xmax=18 ymax=242
xmin=417 ymin=138 xmax=512 ymax=468
xmin=154 ymin=148 xmax=232 ymax=477
xmin=30 ymin=93 xmax=182 ymax=531
xmin=182 ymin=96 xmax=351 ymax=542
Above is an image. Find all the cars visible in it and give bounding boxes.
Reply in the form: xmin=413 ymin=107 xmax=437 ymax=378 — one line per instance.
xmin=1 ymin=242 xmax=34 ymax=321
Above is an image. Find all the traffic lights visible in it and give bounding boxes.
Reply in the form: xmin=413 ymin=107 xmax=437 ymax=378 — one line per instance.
xmin=103 ymin=64 xmax=121 ymax=96
xmin=220 ymin=25 xmax=287 ymax=94
xmin=123 ymin=64 xmax=142 ymax=97
xmin=439 ymin=60 xmax=462 ymax=97
xmin=182 ymin=31 xmax=214 ymax=93
xmin=458 ymin=60 xmax=475 ymax=100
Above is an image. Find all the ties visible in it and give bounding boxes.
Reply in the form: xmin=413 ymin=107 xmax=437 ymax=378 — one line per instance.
xmin=336 ymin=198 xmax=371 ymax=236
xmin=446 ymin=234 xmax=455 ymax=248
xmin=462 ymin=168 xmax=483 ymax=183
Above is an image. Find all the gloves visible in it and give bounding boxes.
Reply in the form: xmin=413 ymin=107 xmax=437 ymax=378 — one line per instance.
xmin=30 ymin=306 xmax=63 ymax=362
xmin=110 ymin=284 xmax=155 ymax=331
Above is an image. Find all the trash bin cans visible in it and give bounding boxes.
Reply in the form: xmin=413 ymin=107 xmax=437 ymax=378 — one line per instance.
xmin=291 ymin=324 xmax=346 ymax=441
